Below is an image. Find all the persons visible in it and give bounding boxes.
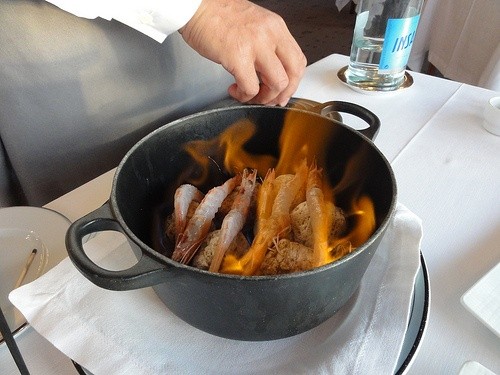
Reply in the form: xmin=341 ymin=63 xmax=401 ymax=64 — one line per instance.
xmin=43 ymin=0 xmax=307 ymax=107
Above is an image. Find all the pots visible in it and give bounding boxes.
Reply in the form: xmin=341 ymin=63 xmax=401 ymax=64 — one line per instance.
xmin=66 ymin=102 xmax=397 ymax=342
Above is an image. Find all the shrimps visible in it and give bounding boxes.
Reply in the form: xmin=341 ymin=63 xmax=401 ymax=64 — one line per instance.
xmin=174 ymin=184 xmax=205 ymax=244
xmin=208 ymin=169 xmax=258 ymax=273
xmin=223 ymin=156 xmax=328 ymax=274
xmin=171 ymin=175 xmax=241 ymax=265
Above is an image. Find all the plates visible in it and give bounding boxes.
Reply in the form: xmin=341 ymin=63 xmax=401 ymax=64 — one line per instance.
xmin=459 ymin=263 xmax=500 ymax=338
xmin=456 ymin=360 xmax=497 ymax=375
xmin=0 ymin=207 xmax=74 ymax=346
xmin=276 ymin=96 xmax=343 ymax=122
xmin=336 ymin=65 xmax=414 ymax=94
xmin=394 ymin=250 xmax=432 ymax=375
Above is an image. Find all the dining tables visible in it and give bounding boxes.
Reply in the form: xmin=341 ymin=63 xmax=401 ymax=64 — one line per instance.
xmin=1 ymin=54 xmax=500 ymax=374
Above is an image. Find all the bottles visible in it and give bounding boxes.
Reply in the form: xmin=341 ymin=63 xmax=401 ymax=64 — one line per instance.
xmin=345 ymin=0 xmax=425 ymax=90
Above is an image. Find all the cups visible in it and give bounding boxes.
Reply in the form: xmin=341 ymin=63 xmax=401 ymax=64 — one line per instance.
xmin=482 ymin=97 xmax=500 ymax=135
